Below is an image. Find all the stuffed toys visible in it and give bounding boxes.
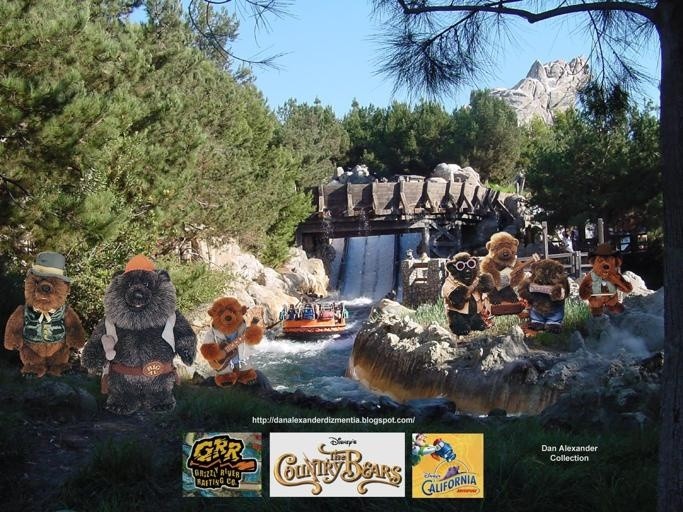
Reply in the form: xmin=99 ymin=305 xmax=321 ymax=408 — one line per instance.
xmin=200 ymin=297 xmax=263 ymax=388
xmin=579 ymin=244 xmax=633 ymax=317
xmin=441 ymin=251 xmax=494 ymax=336
xmin=482 ymin=231 xmax=525 ymax=305
xmin=81 ymin=253 xmax=198 ymax=416
xmin=518 ymin=260 xmax=570 ymax=334
xmin=4 ymin=252 xmax=85 ymax=379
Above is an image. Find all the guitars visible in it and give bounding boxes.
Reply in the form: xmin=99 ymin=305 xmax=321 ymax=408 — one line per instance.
xmin=448 ymin=277 xmax=481 ymax=310
xmin=496 ymin=253 xmax=541 ymax=292
xmin=208 ymin=317 xmax=259 ymax=371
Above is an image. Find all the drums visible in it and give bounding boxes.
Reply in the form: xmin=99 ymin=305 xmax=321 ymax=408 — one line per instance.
xmin=589 ymin=294 xmax=618 ymax=308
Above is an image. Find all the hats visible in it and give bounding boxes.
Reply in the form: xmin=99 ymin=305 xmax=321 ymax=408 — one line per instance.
xmin=589 ymin=243 xmax=619 ymax=256
xmin=123 ymin=253 xmax=155 ymax=273
xmin=27 ymin=252 xmax=71 ymax=283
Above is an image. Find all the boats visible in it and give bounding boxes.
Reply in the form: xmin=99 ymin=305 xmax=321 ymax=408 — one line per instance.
xmin=282 ymin=304 xmax=346 ymax=338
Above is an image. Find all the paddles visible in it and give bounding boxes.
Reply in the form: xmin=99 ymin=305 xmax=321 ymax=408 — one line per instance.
xmin=266 ymin=320 xmax=281 ymax=329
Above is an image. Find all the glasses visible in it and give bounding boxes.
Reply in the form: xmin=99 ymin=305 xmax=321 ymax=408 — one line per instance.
xmin=451 ymin=259 xmax=477 ymax=271
